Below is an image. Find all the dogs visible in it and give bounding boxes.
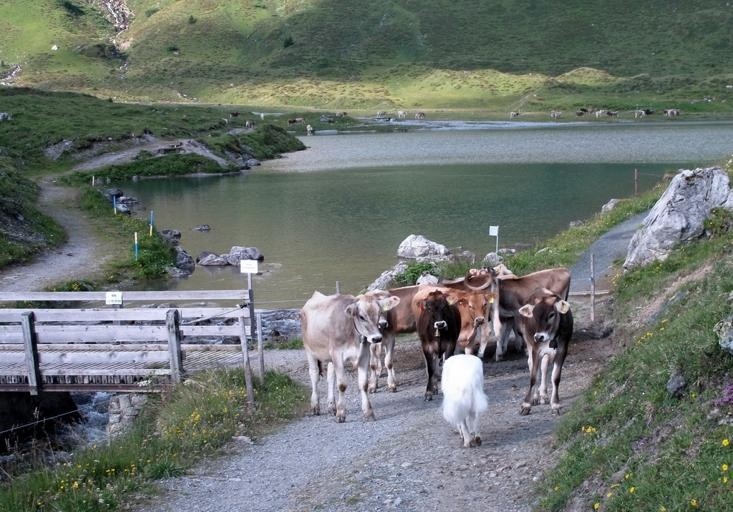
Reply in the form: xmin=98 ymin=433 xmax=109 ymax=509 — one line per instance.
xmin=441 ymin=354 xmax=490 ymax=448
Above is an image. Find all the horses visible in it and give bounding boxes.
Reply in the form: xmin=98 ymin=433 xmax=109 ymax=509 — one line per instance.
xmin=518 ymin=287 xmax=573 ymax=417
xmin=382 ymin=273 xmax=494 ymax=393
xmin=469 ymin=267 xmax=572 ymax=362
xmin=369 ymin=289 xmax=400 ymax=394
xmin=414 ymin=264 xmax=517 ymax=284
xmin=299 ymin=290 xmax=400 ymax=423
xmin=416 ymin=290 xmax=461 ymax=402
